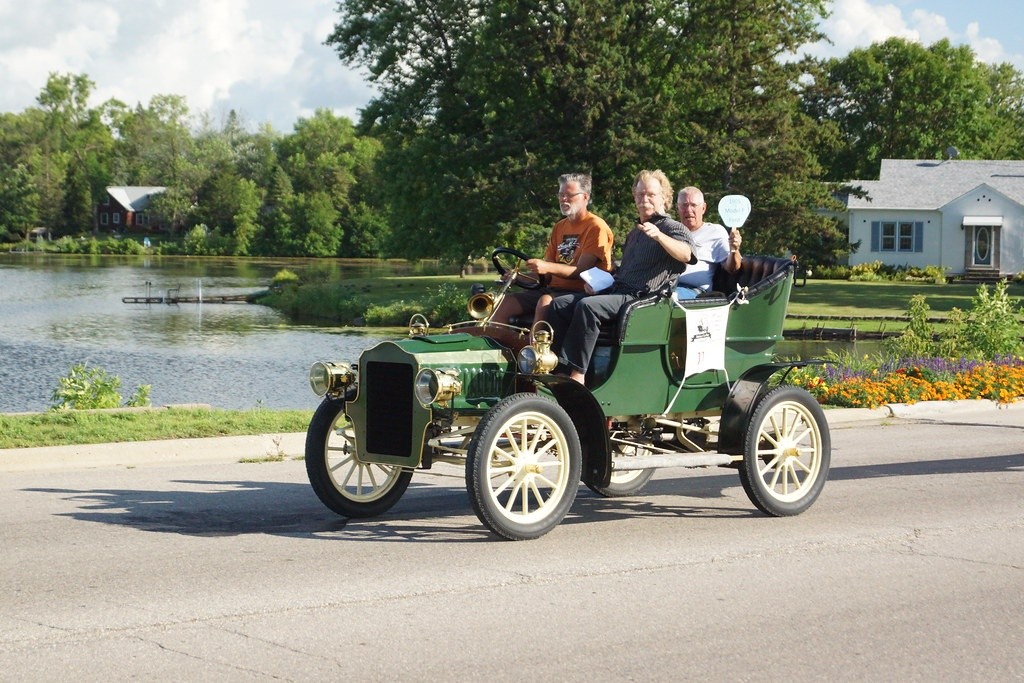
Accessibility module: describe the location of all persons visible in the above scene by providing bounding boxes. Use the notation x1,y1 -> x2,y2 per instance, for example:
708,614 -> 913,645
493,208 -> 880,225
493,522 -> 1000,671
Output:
674,187 -> 742,299
489,173 -> 614,338
545,169 -> 699,386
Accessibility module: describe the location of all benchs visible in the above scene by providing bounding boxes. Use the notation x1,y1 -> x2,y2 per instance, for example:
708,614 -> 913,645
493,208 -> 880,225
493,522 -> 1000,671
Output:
508,274 -> 682,347
712,255 -> 797,300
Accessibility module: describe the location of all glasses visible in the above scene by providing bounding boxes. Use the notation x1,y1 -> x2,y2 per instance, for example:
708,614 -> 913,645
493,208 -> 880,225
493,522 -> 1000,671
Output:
557,193 -> 586,198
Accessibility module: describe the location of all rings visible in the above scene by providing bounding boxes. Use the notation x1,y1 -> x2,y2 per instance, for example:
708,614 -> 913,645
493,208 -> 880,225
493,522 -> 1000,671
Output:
736,238 -> 738,242
